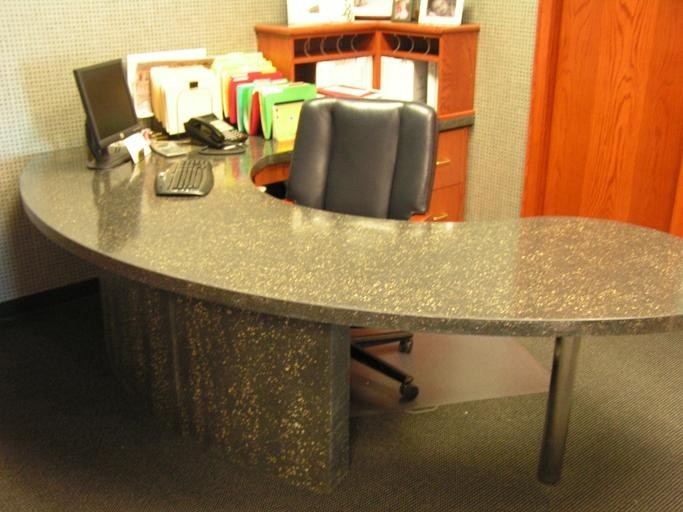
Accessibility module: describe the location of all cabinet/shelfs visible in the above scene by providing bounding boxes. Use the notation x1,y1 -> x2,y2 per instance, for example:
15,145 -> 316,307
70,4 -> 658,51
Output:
255,15 -> 480,117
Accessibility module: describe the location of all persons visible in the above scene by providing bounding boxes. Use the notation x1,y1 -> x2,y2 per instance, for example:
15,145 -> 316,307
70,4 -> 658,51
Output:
427,0 -> 456,18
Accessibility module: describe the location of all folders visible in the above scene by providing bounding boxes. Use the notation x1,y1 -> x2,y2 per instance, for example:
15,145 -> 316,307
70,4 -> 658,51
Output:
208,50 -> 321,141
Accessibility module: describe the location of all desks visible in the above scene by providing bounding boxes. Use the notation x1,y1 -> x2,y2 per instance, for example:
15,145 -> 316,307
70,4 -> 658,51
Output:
18,128 -> 682,493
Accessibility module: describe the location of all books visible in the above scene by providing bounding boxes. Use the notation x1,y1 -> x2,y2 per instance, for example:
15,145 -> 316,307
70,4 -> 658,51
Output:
126,48 -> 383,141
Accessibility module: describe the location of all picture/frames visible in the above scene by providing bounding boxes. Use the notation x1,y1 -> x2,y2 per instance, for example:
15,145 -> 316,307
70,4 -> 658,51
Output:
391,0 -> 465,28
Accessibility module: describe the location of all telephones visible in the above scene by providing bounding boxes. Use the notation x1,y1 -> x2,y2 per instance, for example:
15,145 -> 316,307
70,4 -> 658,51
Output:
184,113 -> 248,149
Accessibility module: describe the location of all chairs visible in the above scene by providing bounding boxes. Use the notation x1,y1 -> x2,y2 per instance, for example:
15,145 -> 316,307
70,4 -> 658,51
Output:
282,95 -> 437,406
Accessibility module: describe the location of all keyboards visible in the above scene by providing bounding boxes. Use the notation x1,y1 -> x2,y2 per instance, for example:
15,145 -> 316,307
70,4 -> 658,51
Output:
155,159 -> 214,196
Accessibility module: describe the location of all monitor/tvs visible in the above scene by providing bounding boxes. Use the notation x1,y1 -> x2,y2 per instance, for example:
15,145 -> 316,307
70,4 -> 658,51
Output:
73,58 -> 143,170
